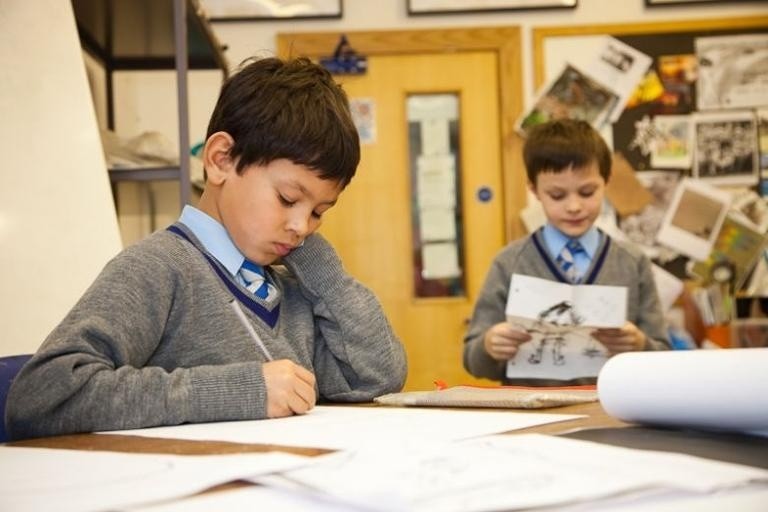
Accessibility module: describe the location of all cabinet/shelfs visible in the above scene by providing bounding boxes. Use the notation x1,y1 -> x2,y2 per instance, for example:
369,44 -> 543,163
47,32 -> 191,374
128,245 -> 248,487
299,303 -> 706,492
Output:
72,0 -> 232,236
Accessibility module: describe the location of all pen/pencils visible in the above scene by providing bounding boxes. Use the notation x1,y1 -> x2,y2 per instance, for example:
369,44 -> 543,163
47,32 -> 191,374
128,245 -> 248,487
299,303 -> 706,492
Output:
228,299 -> 274,362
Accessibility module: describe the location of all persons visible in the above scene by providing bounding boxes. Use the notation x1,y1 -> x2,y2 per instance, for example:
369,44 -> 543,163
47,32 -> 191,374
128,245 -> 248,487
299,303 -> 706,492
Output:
1,40 -> 407,443
462,118 -> 673,387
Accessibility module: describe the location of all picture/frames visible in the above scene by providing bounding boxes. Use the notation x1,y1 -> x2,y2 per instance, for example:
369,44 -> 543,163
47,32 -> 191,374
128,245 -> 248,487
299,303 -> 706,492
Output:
644,0 -> 767,11
196,0 -> 345,24
404,0 -> 580,18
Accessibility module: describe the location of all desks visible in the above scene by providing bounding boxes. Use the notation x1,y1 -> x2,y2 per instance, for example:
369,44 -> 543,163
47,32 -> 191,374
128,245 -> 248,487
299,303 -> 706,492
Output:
0,379 -> 768,511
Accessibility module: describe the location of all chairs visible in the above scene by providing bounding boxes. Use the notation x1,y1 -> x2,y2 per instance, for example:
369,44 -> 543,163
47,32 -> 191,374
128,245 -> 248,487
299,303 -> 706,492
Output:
0,352 -> 34,387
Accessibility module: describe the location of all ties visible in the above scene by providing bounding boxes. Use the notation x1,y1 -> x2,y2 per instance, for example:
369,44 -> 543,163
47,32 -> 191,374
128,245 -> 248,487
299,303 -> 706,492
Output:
554,241 -> 594,284
235,256 -> 282,307
